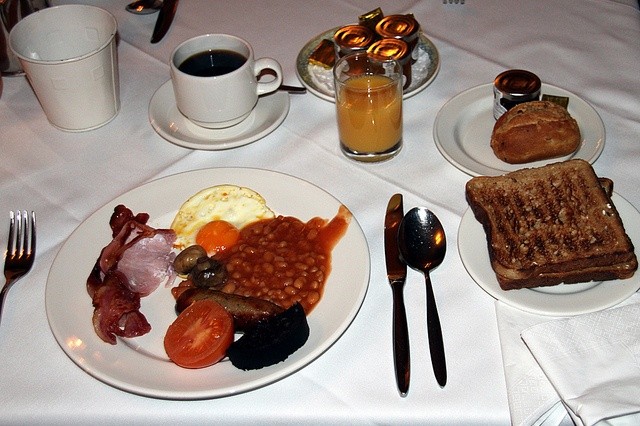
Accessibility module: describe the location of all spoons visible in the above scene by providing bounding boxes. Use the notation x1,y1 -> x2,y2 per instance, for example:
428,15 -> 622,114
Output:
397,206 -> 448,394
125,2 -> 159,16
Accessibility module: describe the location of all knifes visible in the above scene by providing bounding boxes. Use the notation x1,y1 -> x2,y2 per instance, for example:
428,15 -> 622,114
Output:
384,194 -> 411,396
150,1 -> 179,45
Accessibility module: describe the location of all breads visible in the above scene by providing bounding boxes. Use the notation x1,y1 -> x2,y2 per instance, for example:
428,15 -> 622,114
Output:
465,158 -> 634,280
497,175 -> 635,288
492,103 -> 583,165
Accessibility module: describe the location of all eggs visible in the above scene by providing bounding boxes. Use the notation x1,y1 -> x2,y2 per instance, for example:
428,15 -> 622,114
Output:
168,184 -> 276,261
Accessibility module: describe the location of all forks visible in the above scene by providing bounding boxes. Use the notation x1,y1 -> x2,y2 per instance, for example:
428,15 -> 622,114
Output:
1,210 -> 38,320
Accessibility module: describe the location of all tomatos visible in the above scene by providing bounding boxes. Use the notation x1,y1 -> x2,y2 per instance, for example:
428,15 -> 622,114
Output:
163,300 -> 234,369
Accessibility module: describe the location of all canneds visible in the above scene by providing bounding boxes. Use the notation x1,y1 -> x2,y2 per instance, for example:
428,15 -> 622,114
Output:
378,12 -> 421,64
495,68 -> 543,121
366,37 -> 412,90
334,23 -> 376,76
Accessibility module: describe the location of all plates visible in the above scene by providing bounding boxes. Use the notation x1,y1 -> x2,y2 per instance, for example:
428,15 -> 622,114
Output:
458,184 -> 639,317
431,80 -> 606,178
148,77 -> 291,150
44,166 -> 372,400
295,23 -> 440,103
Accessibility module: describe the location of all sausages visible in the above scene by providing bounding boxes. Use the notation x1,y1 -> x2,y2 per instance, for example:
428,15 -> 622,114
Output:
175,288 -> 288,333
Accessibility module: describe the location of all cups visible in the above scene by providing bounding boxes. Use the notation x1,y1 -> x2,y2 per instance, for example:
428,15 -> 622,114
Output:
167,33 -> 283,127
333,52 -> 405,162
8,3 -> 121,132
